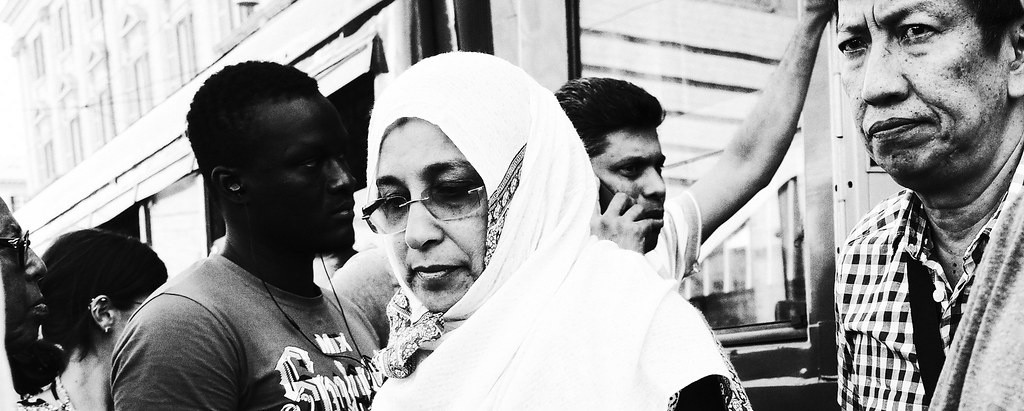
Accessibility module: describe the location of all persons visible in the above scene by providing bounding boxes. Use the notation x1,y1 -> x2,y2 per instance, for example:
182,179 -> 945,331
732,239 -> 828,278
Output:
0,61 -> 399,410
368,50 -> 753,411
834,0 -> 1024,410
556,0 -> 838,291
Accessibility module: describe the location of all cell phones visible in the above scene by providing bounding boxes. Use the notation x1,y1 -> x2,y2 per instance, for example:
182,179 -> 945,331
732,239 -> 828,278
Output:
598,180 -> 644,221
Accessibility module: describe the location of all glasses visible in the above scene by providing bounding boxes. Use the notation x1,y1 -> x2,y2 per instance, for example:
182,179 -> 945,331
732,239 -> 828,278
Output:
0,230 -> 31,270
361,180 -> 487,237
